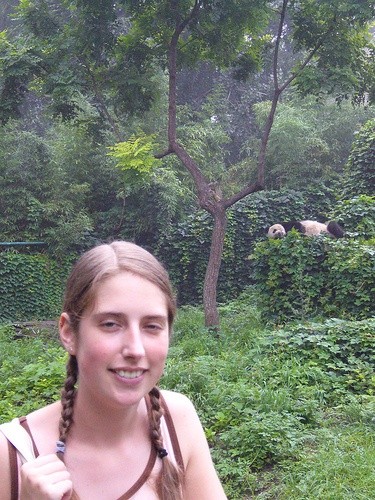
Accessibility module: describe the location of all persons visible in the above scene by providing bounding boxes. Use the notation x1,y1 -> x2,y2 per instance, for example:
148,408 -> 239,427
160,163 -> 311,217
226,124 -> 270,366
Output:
0,240 -> 229,500
